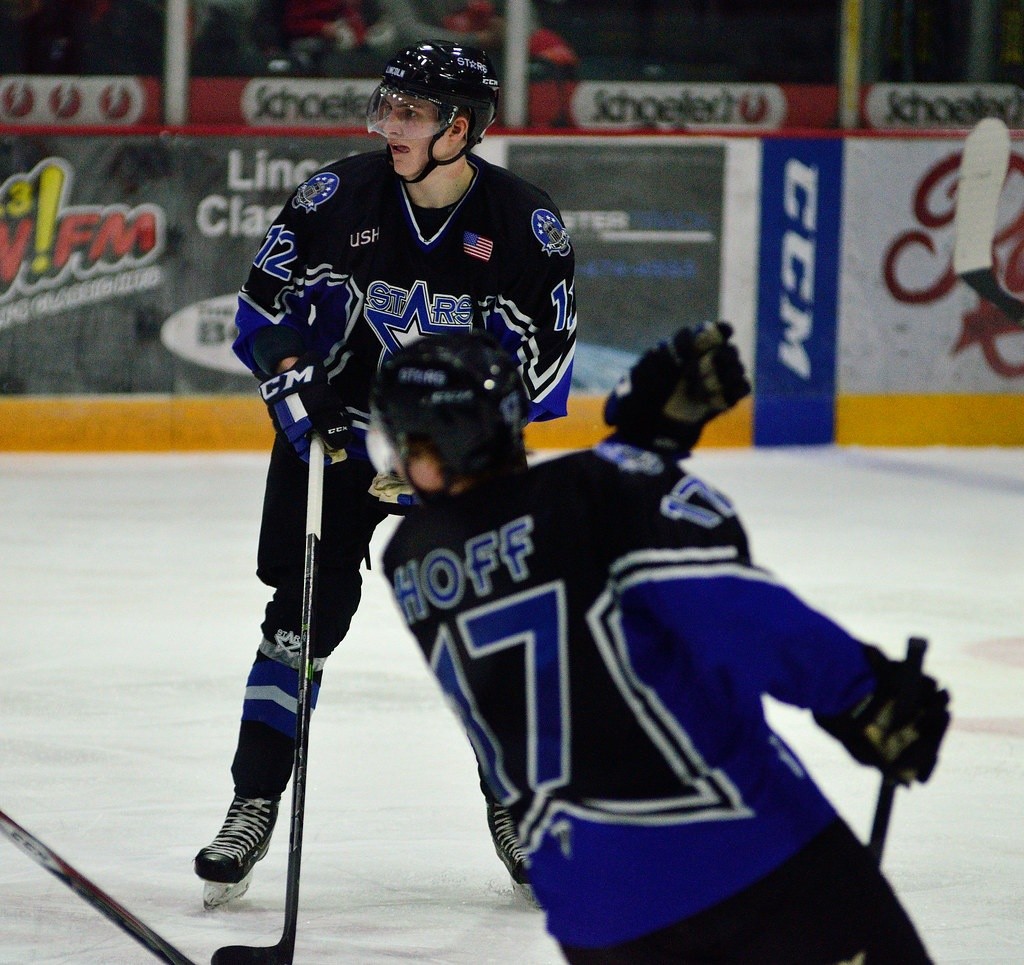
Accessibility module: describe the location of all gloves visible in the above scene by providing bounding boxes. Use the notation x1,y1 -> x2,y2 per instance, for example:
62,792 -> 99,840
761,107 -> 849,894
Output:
604,321 -> 751,457
259,352 -> 353,467
815,647 -> 951,787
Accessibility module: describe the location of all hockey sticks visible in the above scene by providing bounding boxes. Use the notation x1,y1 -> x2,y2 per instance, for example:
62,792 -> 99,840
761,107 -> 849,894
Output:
855,635 -> 933,865
0,811 -> 195,965
208,431 -> 328,965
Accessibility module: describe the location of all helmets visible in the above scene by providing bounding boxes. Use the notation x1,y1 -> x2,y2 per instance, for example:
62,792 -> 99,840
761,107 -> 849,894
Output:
365,38 -> 501,144
366,330 -> 532,479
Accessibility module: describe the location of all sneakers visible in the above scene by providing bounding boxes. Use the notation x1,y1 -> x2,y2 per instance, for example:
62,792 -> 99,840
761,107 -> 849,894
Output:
487,796 -> 545,912
193,793 -> 280,910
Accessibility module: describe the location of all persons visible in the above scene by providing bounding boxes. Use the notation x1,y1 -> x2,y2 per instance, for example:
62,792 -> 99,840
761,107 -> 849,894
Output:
0,1 -> 604,83
376,317 -> 952,963
194,38 -> 579,913
